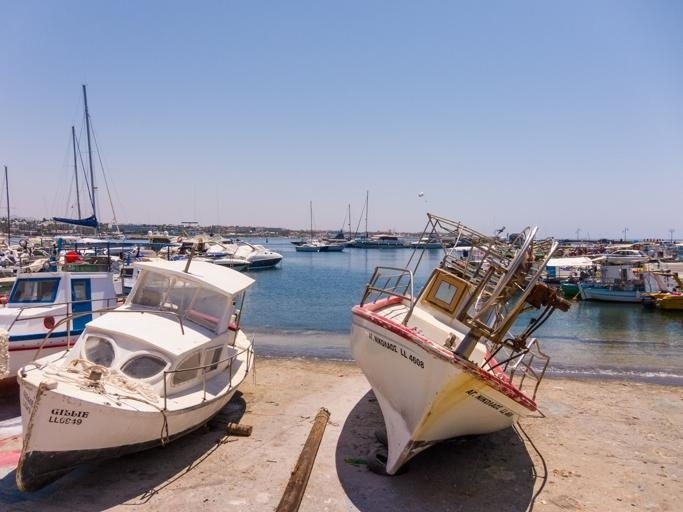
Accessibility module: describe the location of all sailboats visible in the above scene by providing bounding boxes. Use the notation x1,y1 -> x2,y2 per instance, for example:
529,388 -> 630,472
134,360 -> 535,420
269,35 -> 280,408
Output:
289,188 -> 399,252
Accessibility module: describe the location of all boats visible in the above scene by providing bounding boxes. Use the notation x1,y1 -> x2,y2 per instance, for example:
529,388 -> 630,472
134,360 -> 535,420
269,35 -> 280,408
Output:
349,211 -> 571,477
412,232 -> 683,314
